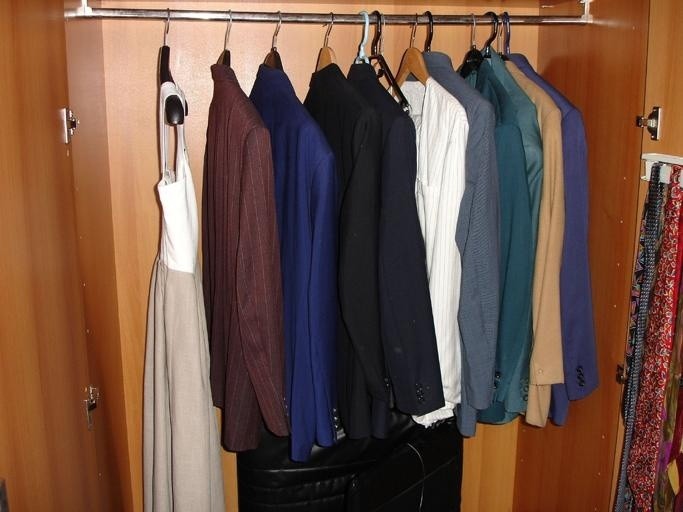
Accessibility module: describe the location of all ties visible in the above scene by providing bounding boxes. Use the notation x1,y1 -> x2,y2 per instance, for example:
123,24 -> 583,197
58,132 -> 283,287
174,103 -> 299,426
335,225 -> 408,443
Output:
615,165 -> 682,512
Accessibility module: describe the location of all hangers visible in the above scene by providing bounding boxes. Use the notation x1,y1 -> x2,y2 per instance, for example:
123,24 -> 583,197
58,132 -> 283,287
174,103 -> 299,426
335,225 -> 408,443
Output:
207,8 -> 512,127
153,7 -> 191,127
